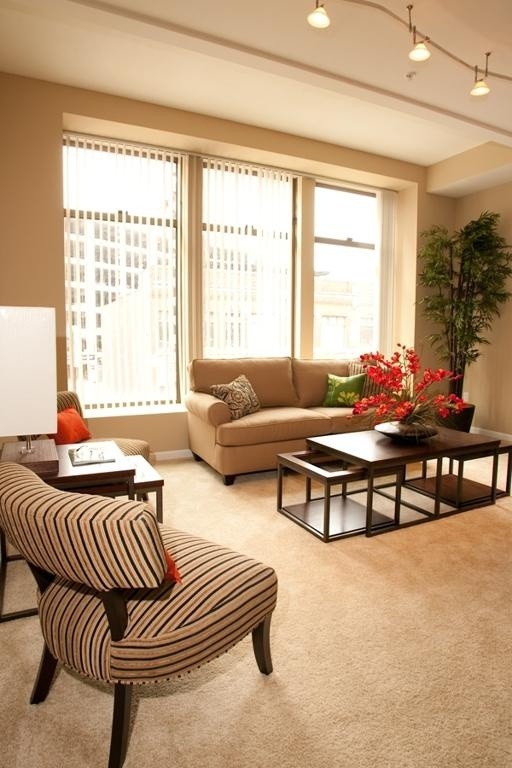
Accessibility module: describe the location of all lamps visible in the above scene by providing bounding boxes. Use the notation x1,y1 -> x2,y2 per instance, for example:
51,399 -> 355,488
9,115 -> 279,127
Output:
1,306 -> 59,473
307,1 -> 329,29
408,27 -> 431,61
470,66 -> 491,96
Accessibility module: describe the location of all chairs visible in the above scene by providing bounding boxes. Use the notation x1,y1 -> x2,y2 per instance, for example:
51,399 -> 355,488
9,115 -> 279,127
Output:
0,460 -> 279,766
17,391 -> 150,459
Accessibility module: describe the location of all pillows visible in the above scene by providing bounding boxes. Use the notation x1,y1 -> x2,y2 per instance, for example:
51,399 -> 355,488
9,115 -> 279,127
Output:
348,363 -> 397,407
321,372 -> 366,407
47,402 -> 94,444
210,374 -> 261,420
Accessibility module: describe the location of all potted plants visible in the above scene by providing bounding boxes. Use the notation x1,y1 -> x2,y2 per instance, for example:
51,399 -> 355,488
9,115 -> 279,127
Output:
413,209 -> 511,433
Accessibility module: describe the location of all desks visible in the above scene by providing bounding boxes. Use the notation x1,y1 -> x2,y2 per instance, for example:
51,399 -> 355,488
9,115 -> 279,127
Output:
0,439 -> 136,624
67,454 -> 164,521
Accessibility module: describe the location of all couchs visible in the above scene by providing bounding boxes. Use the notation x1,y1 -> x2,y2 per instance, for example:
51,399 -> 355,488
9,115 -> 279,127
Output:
182,356 -> 410,485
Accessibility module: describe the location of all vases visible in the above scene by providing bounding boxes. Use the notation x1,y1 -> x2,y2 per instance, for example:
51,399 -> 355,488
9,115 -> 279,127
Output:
374,422 -> 439,448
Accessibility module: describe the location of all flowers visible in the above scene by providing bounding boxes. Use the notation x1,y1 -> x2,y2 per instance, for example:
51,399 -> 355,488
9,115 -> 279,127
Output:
345,343 -> 469,444
